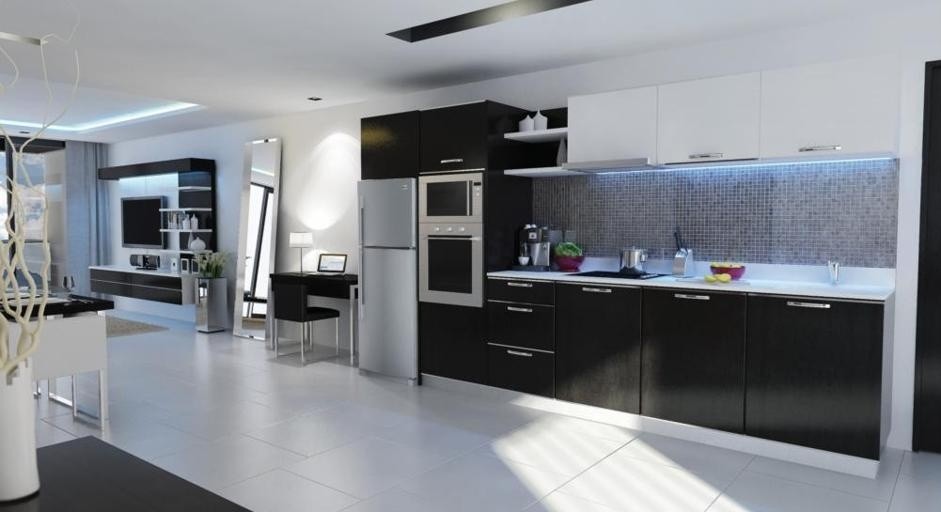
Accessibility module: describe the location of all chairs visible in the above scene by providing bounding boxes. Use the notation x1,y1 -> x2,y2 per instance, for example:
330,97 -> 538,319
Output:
270,279 -> 340,364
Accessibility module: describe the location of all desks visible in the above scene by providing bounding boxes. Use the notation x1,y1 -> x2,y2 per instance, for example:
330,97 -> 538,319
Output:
268,269 -> 360,363
0,434 -> 255,512
1,282 -> 116,446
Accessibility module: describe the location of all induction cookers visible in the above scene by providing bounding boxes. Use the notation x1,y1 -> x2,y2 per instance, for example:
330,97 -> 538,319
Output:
565,270 -> 672,281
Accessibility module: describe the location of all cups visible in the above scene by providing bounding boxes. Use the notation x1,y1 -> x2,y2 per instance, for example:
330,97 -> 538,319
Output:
170,256 -> 178,273
828,262 -> 840,284
518,257 -> 530,266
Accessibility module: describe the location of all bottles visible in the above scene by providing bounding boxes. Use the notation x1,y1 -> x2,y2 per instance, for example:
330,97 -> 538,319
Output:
533,107 -> 548,131
188,233 -> 195,251
518,114 -> 535,133
168,212 -> 198,229
556,137 -> 567,166
191,237 -> 207,252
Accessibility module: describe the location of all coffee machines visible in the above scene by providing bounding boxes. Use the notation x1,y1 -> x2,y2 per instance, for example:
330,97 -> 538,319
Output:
519,227 -> 550,271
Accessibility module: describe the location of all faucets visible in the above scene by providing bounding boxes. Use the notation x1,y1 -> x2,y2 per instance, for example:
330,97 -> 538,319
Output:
833,261 -> 841,282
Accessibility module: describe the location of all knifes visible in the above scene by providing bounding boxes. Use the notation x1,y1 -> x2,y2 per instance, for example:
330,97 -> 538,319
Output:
675,226 -> 689,255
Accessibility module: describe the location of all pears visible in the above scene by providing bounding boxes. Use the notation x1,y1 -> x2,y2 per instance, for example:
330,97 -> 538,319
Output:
704,263 -> 742,282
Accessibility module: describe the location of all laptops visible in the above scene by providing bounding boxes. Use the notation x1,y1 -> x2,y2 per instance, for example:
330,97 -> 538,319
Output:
306,253 -> 347,276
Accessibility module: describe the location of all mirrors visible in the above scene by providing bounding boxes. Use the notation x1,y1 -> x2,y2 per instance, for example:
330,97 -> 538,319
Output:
229,134 -> 285,343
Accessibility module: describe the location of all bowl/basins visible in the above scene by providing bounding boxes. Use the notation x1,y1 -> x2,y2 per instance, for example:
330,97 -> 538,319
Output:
553,256 -> 585,273
711,266 -> 746,281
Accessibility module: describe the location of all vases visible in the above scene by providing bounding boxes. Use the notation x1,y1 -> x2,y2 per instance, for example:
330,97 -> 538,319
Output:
193,275 -> 228,335
180,213 -> 207,251
1,353 -> 43,506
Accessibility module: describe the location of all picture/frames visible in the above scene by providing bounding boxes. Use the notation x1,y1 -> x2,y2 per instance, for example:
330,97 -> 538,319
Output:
317,251 -> 349,273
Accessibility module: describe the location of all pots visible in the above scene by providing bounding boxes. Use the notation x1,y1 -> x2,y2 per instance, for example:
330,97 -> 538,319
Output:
619,245 -> 649,276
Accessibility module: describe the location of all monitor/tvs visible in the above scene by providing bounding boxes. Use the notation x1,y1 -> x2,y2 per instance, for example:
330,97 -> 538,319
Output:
120,195 -> 166,250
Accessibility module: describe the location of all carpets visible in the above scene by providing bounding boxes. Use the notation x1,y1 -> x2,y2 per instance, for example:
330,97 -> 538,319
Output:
104,312 -> 169,340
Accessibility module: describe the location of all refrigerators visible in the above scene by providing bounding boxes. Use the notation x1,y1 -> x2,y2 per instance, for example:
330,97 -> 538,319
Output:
358,176 -> 418,378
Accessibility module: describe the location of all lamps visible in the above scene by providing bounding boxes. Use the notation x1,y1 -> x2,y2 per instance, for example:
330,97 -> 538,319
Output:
288,230 -> 316,274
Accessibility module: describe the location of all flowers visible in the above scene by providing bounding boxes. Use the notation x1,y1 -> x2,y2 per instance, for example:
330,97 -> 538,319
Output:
190,249 -> 229,281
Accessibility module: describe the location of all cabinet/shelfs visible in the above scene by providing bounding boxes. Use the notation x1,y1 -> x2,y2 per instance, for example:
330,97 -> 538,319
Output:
85,263 -> 197,307
636,276 -> 750,449
551,273 -> 642,424
480,264 -> 582,409
743,280 -> 899,474
155,182 -> 215,256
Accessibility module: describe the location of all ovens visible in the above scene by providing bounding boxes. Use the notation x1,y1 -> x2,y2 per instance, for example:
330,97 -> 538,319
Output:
416,171 -> 486,311
